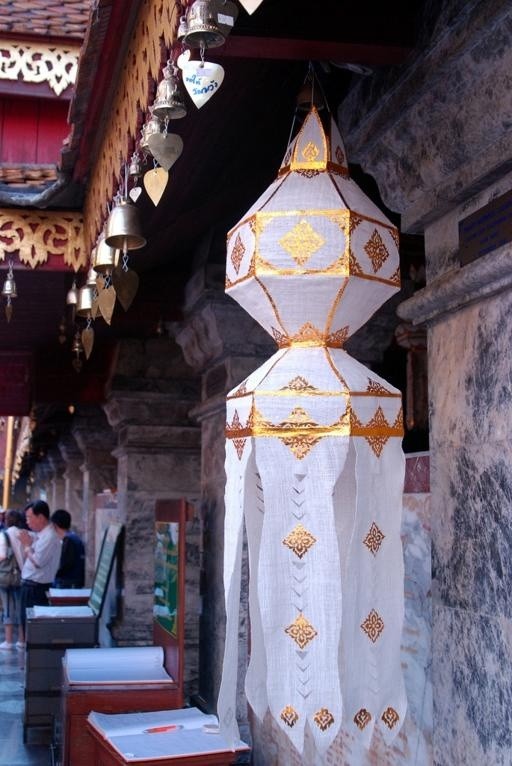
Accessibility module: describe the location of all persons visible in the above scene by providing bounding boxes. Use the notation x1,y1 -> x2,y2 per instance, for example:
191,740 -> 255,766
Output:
0,498 -> 37,649
18,500 -> 60,639
51,510 -> 85,589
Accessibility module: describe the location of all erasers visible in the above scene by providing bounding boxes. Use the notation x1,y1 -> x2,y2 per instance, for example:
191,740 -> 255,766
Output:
203,725 -> 219,734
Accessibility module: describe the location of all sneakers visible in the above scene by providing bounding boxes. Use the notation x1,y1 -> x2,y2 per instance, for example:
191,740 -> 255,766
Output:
0,639 -> 27,650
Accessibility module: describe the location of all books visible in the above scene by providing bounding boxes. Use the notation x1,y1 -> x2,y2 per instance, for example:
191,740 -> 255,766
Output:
61,645 -> 175,685
88,706 -> 253,762
49,588 -> 91,597
33,605 -> 94,619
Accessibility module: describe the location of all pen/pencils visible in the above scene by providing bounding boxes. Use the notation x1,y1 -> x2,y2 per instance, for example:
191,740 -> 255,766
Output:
143,726 -> 183,733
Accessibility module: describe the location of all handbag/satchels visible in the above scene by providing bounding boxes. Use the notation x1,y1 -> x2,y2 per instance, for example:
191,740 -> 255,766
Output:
0,554 -> 21,589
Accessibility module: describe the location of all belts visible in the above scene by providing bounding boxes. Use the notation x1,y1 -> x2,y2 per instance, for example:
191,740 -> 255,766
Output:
20,579 -> 36,586
